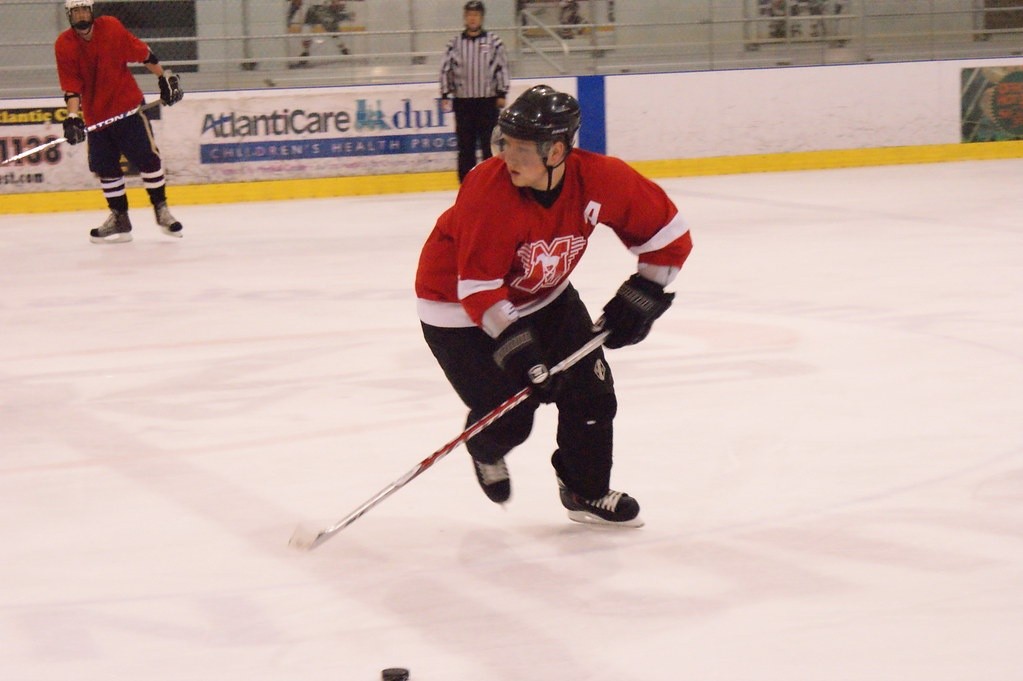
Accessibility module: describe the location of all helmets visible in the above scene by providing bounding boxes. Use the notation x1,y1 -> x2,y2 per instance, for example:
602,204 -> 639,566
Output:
463,2 -> 485,15
500,84 -> 582,143
64,0 -> 95,12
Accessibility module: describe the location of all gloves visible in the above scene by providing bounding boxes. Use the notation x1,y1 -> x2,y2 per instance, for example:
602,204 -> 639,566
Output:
491,319 -> 567,410
62,116 -> 85,144
594,274 -> 675,350
159,69 -> 184,107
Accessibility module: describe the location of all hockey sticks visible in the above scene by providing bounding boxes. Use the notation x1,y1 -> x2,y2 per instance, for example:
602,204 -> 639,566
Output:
0,98 -> 165,166
289,330 -> 614,553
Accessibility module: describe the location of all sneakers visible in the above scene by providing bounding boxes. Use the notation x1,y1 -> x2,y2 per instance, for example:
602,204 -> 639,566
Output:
551,451 -> 645,529
153,201 -> 182,237
90,208 -> 134,244
469,452 -> 512,513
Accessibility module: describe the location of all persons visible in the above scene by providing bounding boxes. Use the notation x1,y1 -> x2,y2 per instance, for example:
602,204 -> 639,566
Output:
411,84 -> 698,527
54,0 -> 187,241
434,0 -> 512,184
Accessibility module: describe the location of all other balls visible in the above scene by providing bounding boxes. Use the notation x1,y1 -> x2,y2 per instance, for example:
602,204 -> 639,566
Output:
382,669 -> 410,681
